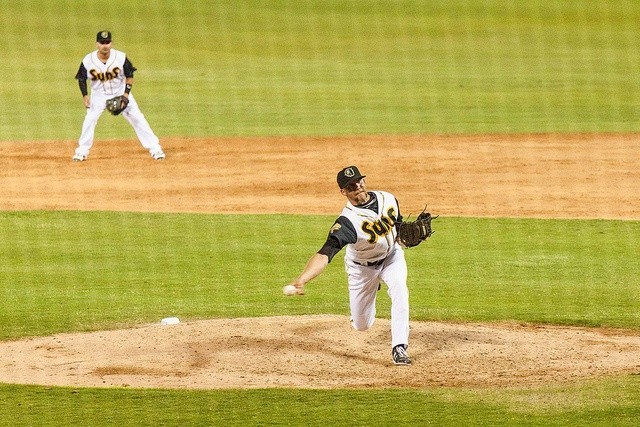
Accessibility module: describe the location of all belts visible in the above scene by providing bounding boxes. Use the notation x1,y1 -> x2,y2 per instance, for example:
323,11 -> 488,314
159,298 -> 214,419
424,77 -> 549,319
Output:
353,258 -> 387,267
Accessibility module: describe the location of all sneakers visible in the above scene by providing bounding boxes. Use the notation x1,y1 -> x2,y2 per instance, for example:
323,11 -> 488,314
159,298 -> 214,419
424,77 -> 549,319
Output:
392,345 -> 412,365
73,153 -> 88,161
153,152 -> 166,160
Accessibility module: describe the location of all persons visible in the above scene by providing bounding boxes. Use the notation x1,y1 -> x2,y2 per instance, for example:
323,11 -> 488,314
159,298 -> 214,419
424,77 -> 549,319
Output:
281,165 -> 439,366
72,30 -> 166,162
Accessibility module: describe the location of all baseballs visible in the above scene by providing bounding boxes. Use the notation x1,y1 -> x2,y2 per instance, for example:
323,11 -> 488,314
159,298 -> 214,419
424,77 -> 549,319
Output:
282,284 -> 297,295
161,316 -> 180,326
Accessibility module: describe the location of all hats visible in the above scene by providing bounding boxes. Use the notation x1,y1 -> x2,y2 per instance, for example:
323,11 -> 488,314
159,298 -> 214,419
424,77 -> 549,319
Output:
337,165 -> 366,188
97,30 -> 112,41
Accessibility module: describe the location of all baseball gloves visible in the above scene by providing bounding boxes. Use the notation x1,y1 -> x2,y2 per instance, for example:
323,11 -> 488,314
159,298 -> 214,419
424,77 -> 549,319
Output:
105,95 -> 129,116
394,205 -> 439,248
